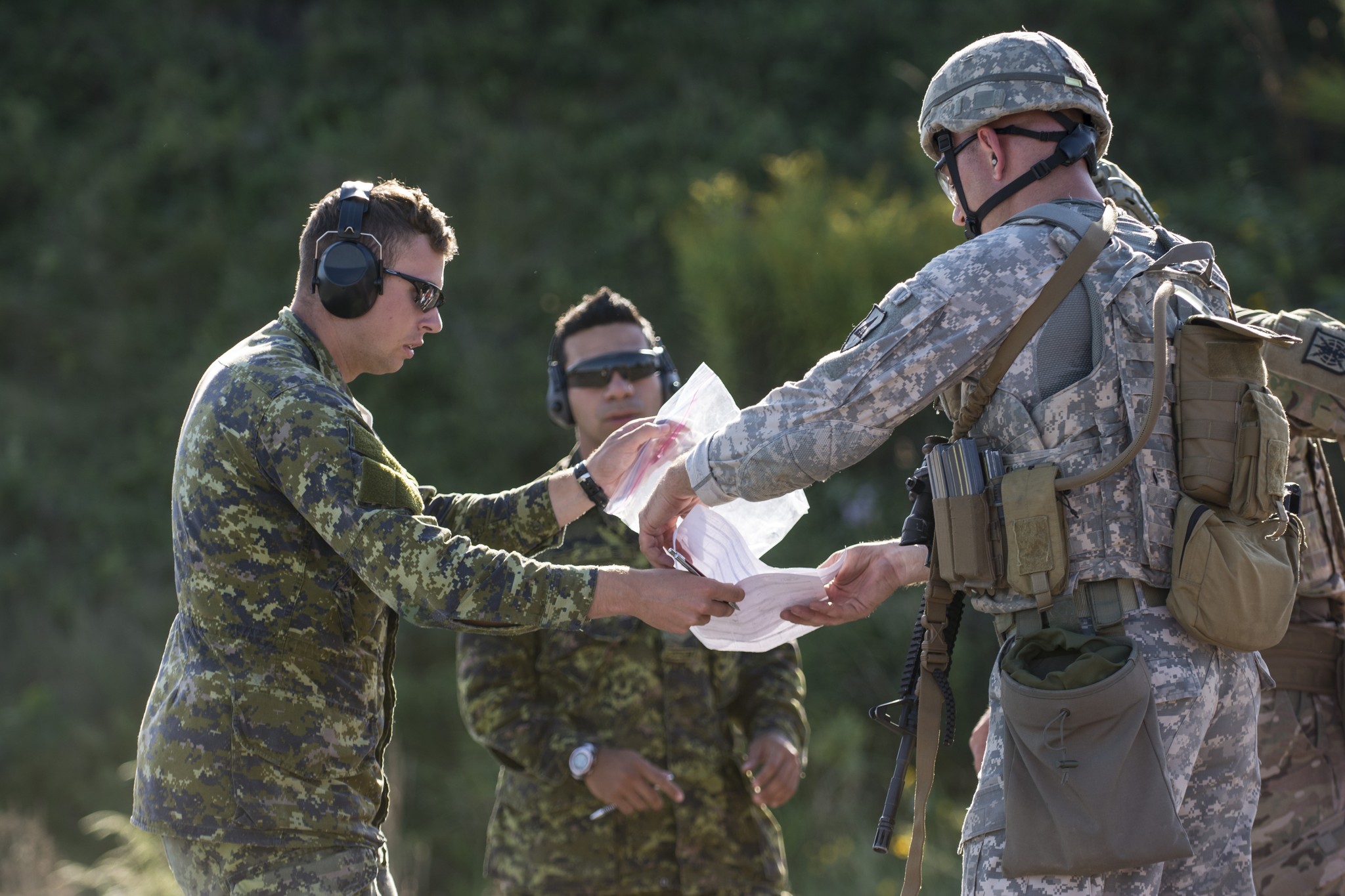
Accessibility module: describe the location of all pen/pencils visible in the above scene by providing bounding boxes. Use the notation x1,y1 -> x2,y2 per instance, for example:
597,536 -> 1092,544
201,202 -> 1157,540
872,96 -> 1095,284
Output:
588,773 -> 673,822
668,547 -> 743,612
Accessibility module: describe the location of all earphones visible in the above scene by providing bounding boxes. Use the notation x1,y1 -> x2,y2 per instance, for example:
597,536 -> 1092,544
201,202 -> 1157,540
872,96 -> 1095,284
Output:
991,153 -> 998,167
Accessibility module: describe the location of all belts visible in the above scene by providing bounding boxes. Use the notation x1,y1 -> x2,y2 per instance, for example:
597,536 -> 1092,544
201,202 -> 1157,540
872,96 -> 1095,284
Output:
991,571 -> 1172,652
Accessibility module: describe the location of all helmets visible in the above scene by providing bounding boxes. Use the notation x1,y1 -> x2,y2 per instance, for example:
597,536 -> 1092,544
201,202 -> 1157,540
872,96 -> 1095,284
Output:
917,29 -> 1114,167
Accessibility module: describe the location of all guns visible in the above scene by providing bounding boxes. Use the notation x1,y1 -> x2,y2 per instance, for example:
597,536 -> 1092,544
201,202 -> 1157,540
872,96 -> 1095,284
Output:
869,435 -> 964,857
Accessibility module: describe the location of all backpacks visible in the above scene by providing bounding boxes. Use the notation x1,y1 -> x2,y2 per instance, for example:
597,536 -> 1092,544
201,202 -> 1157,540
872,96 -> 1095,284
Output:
1000,202 -> 1345,652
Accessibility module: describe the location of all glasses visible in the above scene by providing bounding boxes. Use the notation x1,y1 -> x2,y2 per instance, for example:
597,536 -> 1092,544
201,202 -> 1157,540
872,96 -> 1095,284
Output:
382,267 -> 447,313
934,131 -> 978,207
560,346 -> 663,389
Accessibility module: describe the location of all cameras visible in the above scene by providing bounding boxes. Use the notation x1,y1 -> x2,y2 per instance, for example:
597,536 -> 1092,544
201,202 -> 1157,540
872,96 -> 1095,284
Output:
1284,482 -> 1302,516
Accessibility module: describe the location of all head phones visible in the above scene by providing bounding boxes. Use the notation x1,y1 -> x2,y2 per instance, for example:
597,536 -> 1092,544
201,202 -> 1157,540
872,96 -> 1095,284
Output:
546,302 -> 681,429
311,182 -> 384,318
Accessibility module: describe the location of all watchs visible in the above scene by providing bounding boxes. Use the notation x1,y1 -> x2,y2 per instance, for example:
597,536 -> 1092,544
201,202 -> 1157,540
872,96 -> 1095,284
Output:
568,742 -> 599,786
574,458 -> 609,508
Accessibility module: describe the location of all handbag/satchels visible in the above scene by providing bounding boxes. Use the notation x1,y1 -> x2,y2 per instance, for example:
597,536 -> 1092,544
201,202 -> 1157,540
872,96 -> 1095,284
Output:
1001,628 -> 1197,879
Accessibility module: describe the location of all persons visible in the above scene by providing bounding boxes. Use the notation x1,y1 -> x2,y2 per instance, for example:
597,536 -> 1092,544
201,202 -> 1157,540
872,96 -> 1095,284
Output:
129,184 -> 743,895
453,285 -> 811,896
639,32 -> 1295,896
965,300 -> 1344,896
